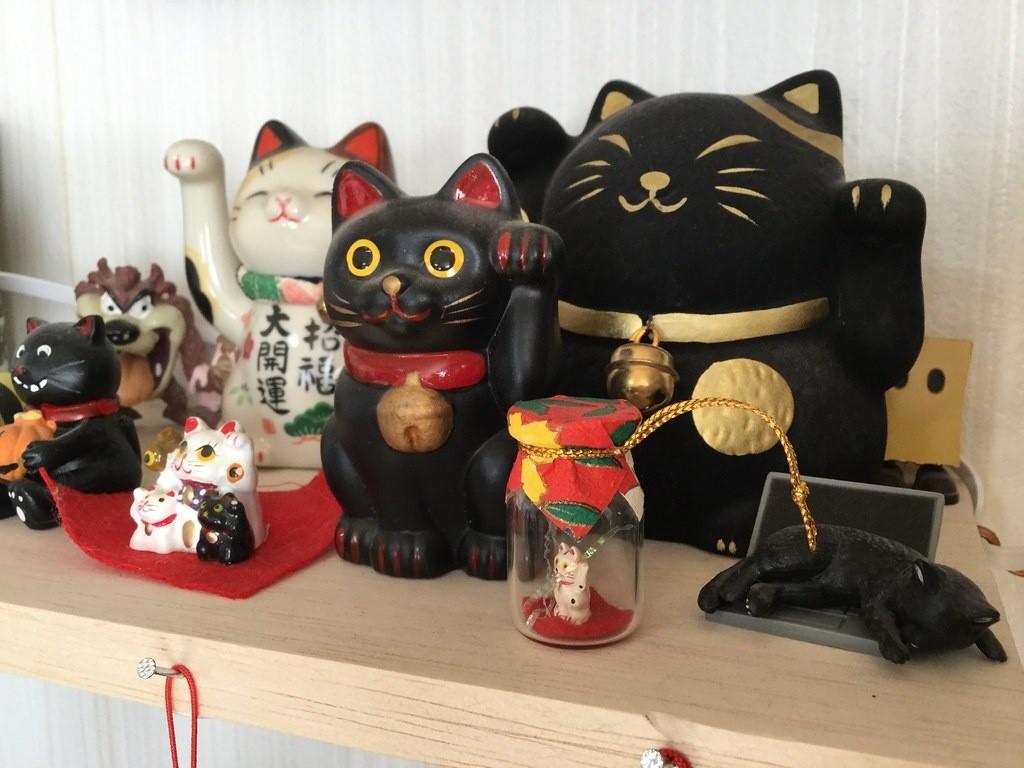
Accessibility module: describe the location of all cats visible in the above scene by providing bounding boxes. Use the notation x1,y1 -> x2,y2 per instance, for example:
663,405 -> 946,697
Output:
196,490 -> 254,565
0,314 -> 144,530
697,522 -> 1010,665
129,486 -> 202,555
153,415 -> 271,549
163,118 -> 397,472
485,69 -> 928,557
320,152 -> 567,579
552,542 -> 591,625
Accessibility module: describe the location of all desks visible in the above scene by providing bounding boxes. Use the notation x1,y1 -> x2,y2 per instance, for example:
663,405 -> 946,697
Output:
0,464 -> 1024,768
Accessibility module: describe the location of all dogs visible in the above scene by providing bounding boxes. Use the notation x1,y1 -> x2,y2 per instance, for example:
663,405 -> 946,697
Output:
73,257 -> 223,429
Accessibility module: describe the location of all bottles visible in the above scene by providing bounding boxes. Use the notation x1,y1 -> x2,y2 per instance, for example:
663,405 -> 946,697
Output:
506,395 -> 640,642
